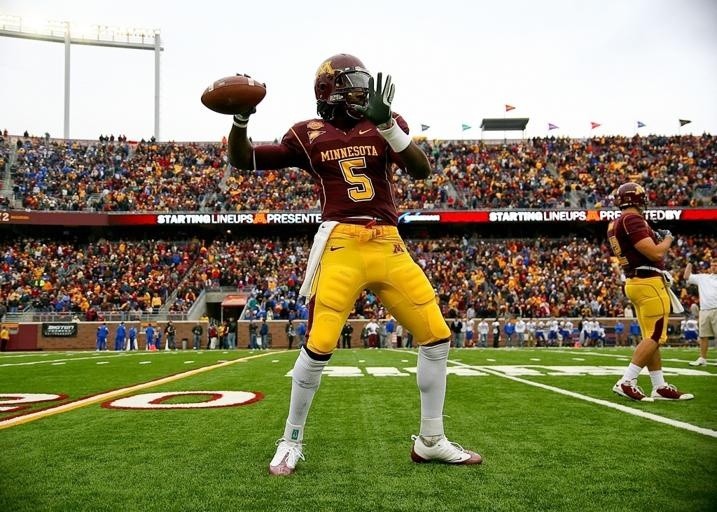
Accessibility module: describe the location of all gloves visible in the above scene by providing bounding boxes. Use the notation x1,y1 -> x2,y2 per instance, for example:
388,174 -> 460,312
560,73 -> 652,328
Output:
687,253 -> 698,264
348,68 -> 396,130
654,229 -> 675,243
231,70 -> 268,130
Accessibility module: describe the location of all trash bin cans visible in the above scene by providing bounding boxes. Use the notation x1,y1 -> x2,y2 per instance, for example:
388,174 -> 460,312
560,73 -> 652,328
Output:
182,338 -> 188,350
256,336 -> 261,345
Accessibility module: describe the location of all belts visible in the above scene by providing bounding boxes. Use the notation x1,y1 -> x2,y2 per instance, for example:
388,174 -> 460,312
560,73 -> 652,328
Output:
332,224 -> 399,242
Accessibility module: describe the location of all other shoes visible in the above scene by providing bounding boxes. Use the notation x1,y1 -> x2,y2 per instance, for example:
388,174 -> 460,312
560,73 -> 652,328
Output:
452,344 -> 633,348
221,348 -> 270,351
95,346 -> 196,351
364,346 -> 413,349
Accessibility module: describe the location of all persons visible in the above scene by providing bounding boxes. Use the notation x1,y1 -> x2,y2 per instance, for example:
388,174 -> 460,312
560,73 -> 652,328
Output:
0,129 -> 717,214
684,253 -> 717,367
607,182 -> 694,403
1,234 -> 717,351
227,54 -> 483,474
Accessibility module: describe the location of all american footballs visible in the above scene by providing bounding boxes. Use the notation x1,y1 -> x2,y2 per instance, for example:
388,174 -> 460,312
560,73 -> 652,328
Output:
200,76 -> 266,114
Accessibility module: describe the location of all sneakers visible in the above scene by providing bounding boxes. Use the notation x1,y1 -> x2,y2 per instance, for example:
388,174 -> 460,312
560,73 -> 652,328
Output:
651,382 -> 695,402
611,377 -> 655,403
688,357 -> 710,366
409,433 -> 482,466
267,434 -> 306,478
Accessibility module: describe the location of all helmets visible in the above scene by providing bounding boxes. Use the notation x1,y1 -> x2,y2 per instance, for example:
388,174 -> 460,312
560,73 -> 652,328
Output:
614,182 -> 649,211
313,53 -> 374,124
